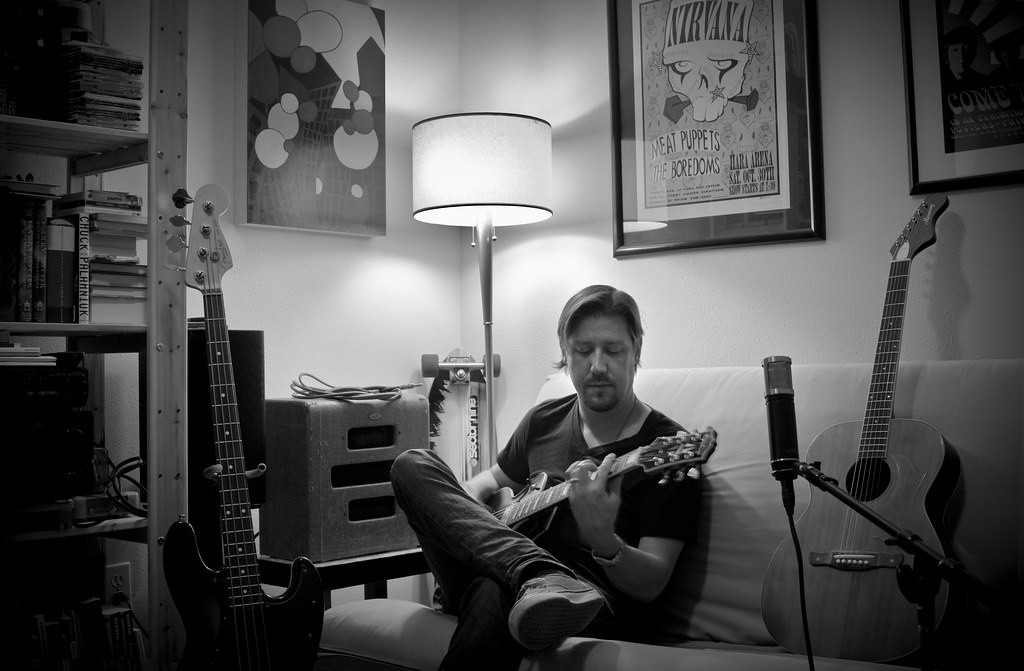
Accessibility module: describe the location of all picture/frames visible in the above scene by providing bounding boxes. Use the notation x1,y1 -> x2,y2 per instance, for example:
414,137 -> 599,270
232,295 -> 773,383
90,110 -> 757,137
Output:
899,0 -> 1024,197
604,0 -> 827,258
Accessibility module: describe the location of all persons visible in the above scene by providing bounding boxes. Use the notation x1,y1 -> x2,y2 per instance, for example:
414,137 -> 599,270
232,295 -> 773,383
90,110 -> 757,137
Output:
390,285 -> 705,671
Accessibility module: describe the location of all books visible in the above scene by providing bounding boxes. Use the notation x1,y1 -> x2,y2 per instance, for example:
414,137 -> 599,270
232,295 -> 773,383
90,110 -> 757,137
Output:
20,597 -> 147,671
0,331 -> 68,366
61,30 -> 144,131
0,181 -> 149,325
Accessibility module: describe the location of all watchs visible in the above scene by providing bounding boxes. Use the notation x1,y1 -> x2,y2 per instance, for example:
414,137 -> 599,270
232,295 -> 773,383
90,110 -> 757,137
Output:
592,533 -> 625,566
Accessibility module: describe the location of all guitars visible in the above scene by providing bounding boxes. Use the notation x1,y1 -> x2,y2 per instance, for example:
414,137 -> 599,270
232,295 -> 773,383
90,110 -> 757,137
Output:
161,181 -> 323,671
486,424 -> 718,527
761,192 -> 963,660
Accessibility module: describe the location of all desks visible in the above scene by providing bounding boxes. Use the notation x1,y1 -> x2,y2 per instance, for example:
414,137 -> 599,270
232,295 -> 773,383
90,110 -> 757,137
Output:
255,545 -> 431,612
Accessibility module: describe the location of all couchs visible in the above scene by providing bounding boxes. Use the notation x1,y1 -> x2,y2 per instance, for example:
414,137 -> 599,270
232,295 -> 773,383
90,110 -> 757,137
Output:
316,358 -> 1024,671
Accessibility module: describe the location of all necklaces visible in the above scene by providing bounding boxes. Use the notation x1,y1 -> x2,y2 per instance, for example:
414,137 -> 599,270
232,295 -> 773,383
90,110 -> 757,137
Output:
615,395 -> 637,441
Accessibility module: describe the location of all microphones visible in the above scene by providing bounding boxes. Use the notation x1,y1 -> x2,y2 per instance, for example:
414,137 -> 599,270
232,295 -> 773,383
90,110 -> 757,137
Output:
762,356 -> 800,515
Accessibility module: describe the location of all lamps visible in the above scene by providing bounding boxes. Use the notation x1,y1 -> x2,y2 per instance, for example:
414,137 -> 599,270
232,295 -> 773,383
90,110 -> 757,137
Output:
410,111 -> 552,474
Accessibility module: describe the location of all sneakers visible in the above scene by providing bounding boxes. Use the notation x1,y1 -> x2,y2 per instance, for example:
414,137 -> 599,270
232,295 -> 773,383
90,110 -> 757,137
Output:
507,569 -> 605,650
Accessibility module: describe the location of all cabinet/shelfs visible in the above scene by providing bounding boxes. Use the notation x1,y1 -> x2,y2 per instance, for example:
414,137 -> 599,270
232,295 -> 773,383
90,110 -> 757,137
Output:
0,0 -> 189,671
